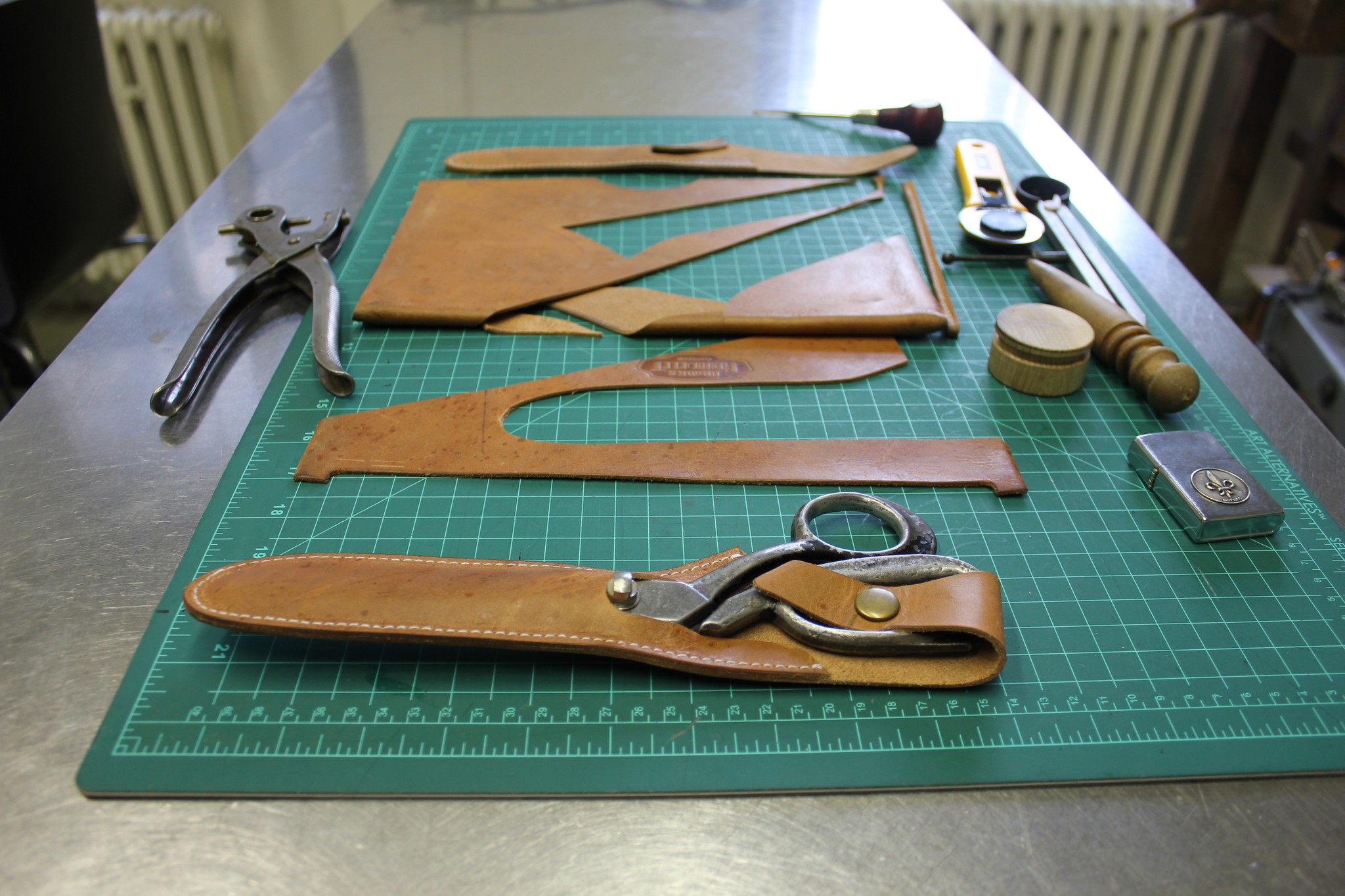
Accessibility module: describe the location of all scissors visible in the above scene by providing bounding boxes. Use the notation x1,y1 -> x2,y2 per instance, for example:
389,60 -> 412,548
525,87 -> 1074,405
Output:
182,490 -> 992,663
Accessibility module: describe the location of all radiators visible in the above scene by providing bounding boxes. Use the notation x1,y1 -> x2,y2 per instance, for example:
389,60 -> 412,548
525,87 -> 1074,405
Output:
944,0 -> 1226,247
39,6 -> 236,314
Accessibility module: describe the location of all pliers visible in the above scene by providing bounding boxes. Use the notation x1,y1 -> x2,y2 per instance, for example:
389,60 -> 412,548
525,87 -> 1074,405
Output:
147,200 -> 357,419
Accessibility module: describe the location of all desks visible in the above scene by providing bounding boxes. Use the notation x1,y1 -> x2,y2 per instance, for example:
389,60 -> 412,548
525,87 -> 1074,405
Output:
0,0 -> 1345,896
